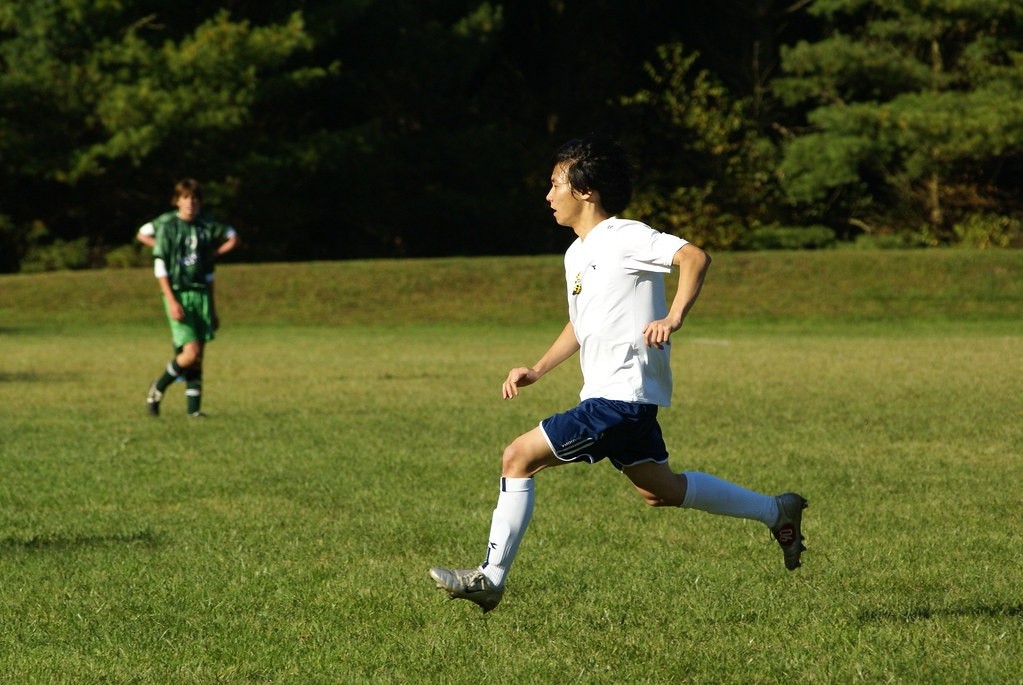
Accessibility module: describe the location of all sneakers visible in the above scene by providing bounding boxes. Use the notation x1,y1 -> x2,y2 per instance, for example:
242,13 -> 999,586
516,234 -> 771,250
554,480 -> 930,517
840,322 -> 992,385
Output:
147,382 -> 162,417
429,567 -> 506,614
191,411 -> 209,420
770,493 -> 809,571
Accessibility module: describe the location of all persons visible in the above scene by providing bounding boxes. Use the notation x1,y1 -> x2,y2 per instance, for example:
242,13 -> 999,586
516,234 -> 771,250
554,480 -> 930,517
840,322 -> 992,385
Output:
136,177 -> 236,420
429,144 -> 809,613
